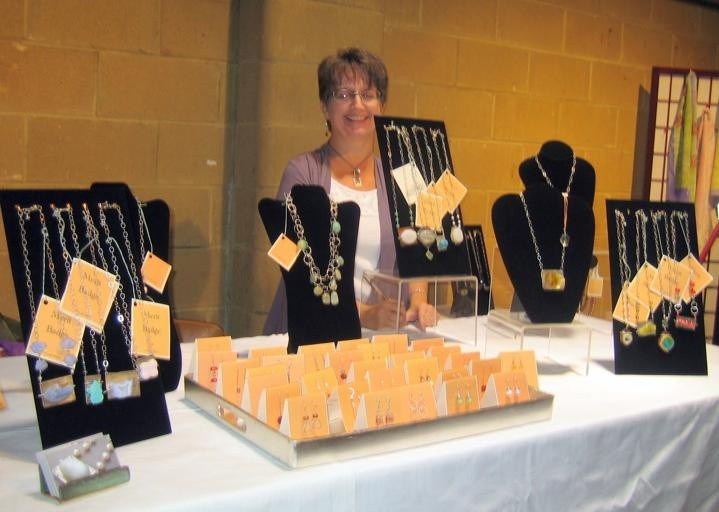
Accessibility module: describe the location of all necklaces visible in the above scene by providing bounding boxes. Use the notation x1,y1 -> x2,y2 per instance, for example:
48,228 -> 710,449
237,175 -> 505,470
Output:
616,208 -> 700,353
282,191 -> 344,308
534,151 -> 578,195
327,143 -> 375,188
17,201 -> 155,406
382,120 -> 491,297
518,193 -> 571,292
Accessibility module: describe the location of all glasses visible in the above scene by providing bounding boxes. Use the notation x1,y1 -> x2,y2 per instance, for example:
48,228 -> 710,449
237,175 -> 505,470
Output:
327,88 -> 382,102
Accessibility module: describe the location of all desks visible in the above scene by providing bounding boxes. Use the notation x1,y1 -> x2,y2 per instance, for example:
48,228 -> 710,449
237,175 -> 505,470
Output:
0,310 -> 719,512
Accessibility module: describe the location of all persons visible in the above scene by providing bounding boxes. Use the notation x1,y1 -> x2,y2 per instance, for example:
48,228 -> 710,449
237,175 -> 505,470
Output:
260,45 -> 443,336
